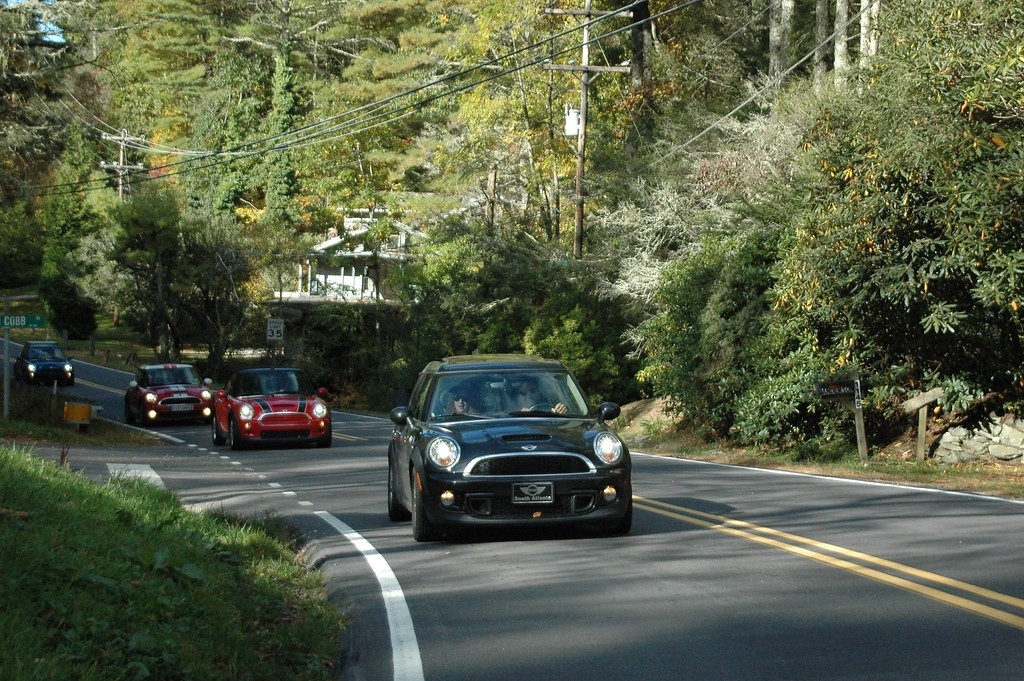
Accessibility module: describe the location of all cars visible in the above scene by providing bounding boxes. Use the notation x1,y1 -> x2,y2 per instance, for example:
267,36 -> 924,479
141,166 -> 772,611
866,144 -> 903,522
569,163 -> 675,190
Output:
211,368 -> 332,446
14,341 -> 74,388
124,363 -> 215,427
387,352 -> 634,541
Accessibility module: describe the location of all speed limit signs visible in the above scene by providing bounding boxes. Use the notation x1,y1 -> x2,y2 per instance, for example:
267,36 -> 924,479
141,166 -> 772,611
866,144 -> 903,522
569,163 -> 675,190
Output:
266,319 -> 284,339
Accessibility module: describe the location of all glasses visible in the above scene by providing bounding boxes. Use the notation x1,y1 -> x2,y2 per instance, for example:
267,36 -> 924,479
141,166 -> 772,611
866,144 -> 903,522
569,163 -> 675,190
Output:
520,387 -> 536,395
453,395 -> 470,402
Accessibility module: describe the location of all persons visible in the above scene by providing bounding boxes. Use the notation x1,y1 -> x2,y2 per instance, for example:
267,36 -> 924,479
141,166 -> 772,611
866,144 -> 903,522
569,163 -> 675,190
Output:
445,384 -> 480,415
506,377 -> 568,415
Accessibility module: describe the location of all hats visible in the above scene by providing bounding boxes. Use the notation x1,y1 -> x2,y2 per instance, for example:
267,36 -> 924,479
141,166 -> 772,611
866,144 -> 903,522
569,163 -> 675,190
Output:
449,384 -> 472,394
